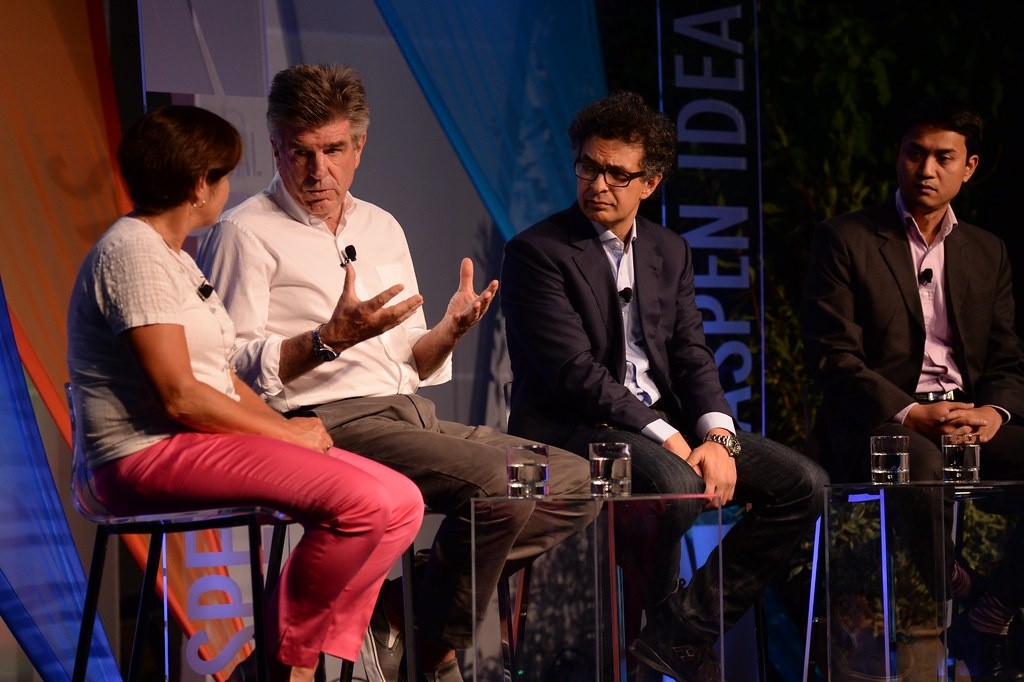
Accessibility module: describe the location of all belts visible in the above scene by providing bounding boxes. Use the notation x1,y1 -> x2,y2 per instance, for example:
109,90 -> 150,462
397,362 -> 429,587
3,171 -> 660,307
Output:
914,389 -> 965,403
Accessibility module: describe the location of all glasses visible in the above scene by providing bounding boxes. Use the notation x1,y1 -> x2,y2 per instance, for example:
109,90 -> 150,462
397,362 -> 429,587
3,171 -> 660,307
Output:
573,158 -> 646,187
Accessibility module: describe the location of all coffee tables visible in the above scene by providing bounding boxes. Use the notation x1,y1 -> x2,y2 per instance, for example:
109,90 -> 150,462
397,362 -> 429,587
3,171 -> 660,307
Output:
473,493 -> 726,682
823,481 -> 1024,682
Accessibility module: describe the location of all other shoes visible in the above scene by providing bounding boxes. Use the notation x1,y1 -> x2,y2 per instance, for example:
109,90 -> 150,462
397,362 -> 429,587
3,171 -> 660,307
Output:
628,621 -> 724,681
423,658 -> 465,682
369,579 -> 405,682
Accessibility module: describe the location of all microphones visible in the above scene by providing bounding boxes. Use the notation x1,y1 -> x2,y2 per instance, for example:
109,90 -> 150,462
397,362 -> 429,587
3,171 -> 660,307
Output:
198,284 -> 213,299
621,288 -> 632,303
923,268 -> 934,283
345,245 -> 356,261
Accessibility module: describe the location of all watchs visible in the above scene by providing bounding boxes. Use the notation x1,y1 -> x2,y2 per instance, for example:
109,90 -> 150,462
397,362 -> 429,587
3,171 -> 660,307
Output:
313,323 -> 340,362
703,434 -> 741,456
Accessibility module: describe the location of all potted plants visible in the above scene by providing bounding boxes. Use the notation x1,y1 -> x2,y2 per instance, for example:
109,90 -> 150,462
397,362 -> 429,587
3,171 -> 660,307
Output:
831,498 -> 1007,682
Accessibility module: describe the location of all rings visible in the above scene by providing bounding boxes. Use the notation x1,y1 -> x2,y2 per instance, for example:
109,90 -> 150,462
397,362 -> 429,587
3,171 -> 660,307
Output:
324,444 -> 330,452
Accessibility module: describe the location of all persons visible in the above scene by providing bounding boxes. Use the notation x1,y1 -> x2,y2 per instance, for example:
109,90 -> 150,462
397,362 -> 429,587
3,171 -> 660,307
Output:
195,63 -> 603,682
810,100 -> 1023,682
66,104 -> 425,682
498,95 -> 829,682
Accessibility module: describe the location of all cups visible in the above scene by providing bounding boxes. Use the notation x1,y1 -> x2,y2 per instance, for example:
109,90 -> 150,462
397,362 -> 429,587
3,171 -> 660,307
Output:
505,444 -> 549,496
942,434 -> 979,481
870,436 -> 909,485
589,443 -> 631,497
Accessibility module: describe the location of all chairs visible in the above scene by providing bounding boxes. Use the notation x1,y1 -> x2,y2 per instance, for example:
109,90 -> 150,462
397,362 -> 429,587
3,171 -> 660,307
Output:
63,380 -> 298,682
